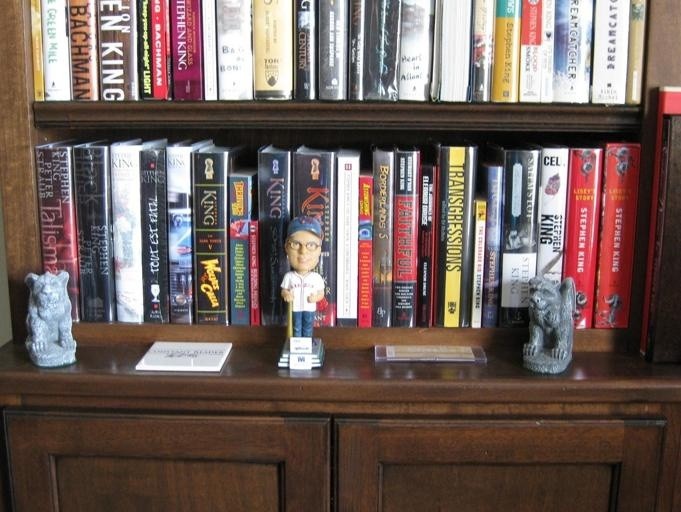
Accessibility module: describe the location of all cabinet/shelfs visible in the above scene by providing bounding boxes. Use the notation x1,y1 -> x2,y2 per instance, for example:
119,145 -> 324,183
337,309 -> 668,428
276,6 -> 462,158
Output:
0,0 -> 681,512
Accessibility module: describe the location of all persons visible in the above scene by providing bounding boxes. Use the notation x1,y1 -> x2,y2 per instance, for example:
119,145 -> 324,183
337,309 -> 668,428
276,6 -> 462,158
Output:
278,214 -> 328,351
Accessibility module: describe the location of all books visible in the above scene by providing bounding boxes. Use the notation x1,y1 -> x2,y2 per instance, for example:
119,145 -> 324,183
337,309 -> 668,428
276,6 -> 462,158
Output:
373,345 -> 489,364
136,340 -> 234,374
641,114 -> 681,360
638,83 -> 681,356
30,0 -> 647,105
37,132 -> 641,329
